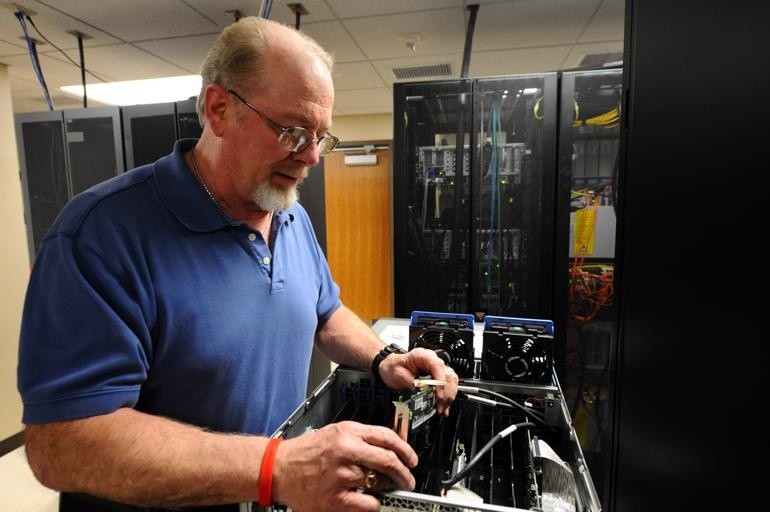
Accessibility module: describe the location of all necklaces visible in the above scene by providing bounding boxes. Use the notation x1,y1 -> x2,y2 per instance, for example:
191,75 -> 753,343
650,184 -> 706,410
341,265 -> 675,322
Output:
187,148 -> 275,240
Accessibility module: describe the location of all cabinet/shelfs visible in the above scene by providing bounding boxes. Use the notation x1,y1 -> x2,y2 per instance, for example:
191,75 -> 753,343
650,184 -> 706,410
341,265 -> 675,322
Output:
299,156 -> 327,262
391,68 -> 627,511
13,101 -> 202,267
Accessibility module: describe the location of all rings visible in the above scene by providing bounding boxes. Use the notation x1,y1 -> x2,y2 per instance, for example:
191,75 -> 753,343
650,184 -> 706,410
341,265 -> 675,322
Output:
363,466 -> 379,491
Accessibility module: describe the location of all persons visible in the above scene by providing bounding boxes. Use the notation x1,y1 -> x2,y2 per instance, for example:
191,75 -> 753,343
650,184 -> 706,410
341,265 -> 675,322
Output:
14,14 -> 461,512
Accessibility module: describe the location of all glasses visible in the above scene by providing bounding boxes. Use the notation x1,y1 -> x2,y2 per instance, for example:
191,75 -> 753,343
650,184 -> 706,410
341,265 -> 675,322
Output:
225,87 -> 339,157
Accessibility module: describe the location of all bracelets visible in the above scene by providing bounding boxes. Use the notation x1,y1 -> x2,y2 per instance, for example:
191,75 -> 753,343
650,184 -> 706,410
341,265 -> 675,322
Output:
258,435 -> 286,510
371,342 -> 407,391
